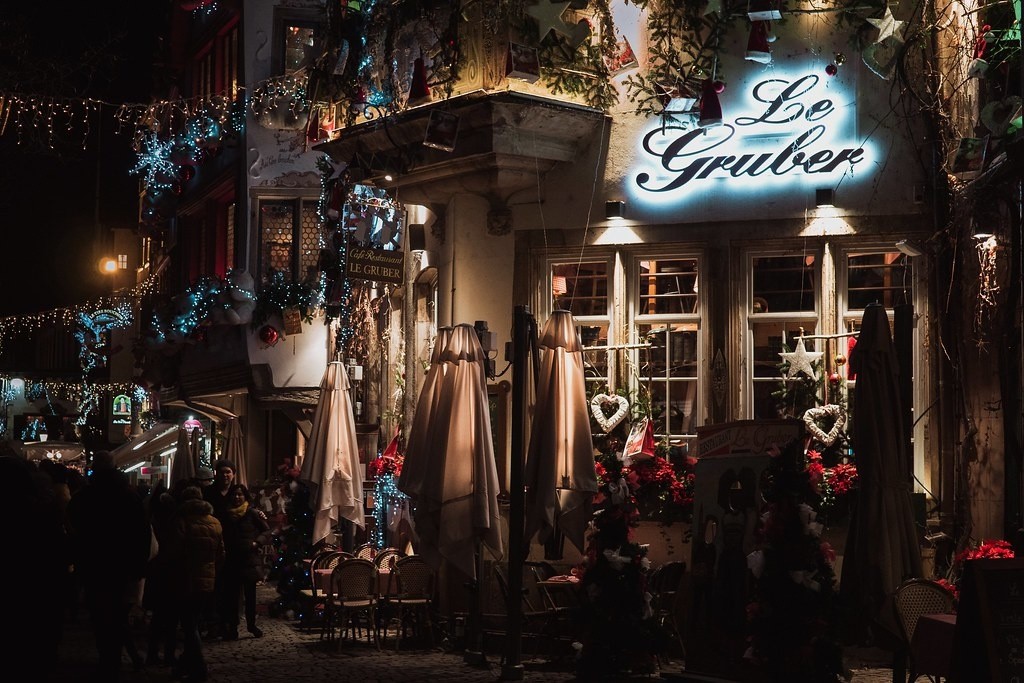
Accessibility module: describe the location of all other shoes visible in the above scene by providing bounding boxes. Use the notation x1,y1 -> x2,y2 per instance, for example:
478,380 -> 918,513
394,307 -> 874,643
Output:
180,671 -> 208,683
135,659 -> 145,672
145,656 -> 164,667
248,626 -> 262,637
163,657 -> 180,665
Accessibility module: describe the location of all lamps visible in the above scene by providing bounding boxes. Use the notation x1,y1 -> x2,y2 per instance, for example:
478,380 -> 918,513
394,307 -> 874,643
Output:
606,202 -> 627,219
816,188 -> 837,210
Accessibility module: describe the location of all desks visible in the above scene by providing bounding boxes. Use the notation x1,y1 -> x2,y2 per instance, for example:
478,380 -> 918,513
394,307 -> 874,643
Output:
315,567 -> 397,641
535,577 -> 589,667
303,556 -> 374,635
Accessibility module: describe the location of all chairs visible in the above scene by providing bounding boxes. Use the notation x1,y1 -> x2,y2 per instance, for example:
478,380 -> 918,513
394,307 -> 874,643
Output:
327,559 -> 383,650
372,548 -> 410,637
891,578 -> 960,683
380,554 -> 434,648
321,552 -> 364,638
300,543 -> 337,633
647,558 -> 686,669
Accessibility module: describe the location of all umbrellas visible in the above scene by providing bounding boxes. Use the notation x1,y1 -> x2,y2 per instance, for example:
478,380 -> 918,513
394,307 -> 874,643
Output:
494,309 -> 598,555
171,427 -> 200,492
841,302 -> 925,640
299,361 -> 365,549
397,323 -> 504,608
221,419 -> 248,490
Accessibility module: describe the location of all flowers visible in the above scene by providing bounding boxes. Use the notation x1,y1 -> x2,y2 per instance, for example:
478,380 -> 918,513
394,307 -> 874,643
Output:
593,444 -> 1015,603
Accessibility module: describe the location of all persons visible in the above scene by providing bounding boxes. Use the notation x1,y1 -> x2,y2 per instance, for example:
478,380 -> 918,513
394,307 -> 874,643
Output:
0,440 -> 271,683
